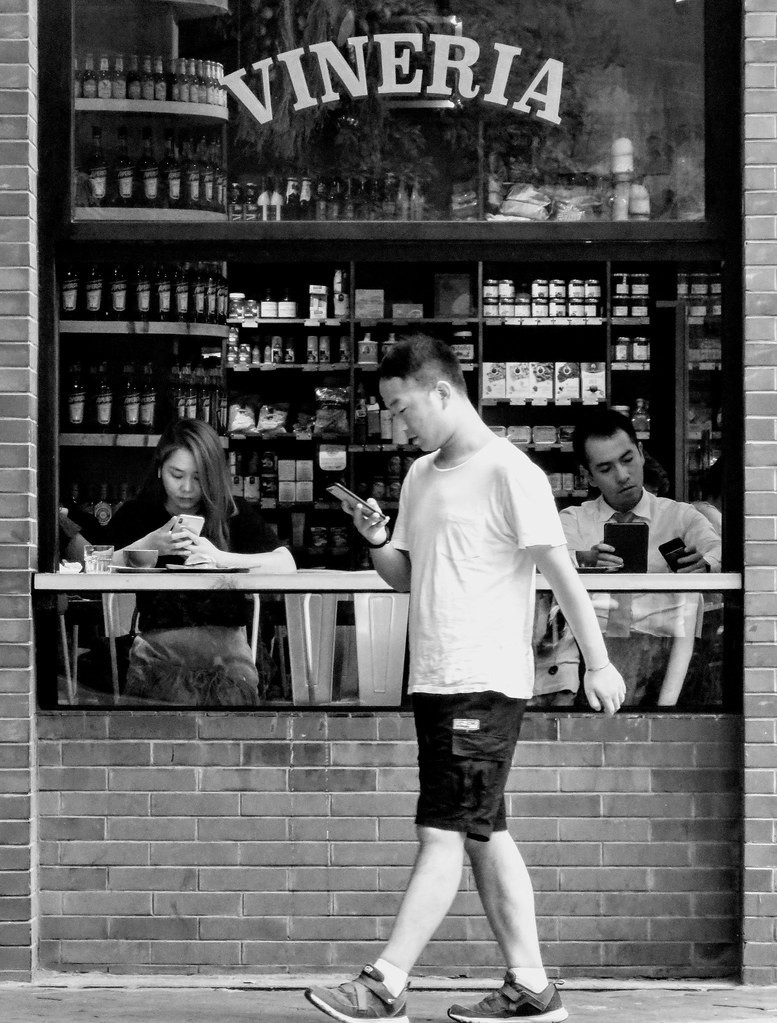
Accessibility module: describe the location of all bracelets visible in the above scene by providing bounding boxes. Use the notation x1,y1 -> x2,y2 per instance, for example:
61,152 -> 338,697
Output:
584,661 -> 611,672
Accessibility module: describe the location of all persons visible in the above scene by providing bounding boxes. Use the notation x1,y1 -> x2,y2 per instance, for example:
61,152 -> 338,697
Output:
303,337 -> 628,1023
557,408 -> 720,706
57,511 -> 94,576
100,415 -> 297,708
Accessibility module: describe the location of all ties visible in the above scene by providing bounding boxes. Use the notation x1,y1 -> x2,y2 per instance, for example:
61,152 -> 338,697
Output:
605,510 -> 638,638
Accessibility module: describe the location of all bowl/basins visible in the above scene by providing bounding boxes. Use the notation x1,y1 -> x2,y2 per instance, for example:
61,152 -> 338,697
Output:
123,550 -> 159,568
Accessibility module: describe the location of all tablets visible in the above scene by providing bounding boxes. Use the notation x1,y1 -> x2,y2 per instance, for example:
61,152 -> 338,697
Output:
603,522 -> 647,574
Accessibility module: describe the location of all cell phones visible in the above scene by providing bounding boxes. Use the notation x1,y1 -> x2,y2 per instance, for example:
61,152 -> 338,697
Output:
171,514 -> 205,541
327,483 -> 386,523
659,537 -> 695,573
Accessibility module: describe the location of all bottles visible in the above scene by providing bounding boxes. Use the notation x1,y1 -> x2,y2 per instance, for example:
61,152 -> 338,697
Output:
76,121 -> 421,218
630,397 -> 650,432
74,52 -> 228,105
59,257 -> 350,325
575,465 -> 588,490
62,338 -> 406,515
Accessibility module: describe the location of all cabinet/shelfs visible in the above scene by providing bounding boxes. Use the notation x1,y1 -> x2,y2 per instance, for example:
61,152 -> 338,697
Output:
56,0 -> 729,614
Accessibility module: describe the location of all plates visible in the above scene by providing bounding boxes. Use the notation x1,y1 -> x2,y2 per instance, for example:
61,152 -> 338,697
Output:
116,565 -> 167,573
576,566 -> 620,573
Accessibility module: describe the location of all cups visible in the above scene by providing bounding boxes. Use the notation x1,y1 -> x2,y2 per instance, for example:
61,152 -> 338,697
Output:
83,545 -> 114,574
548,472 -> 574,490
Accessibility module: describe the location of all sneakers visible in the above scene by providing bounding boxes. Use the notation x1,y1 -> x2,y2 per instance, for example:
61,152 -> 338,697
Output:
446,969 -> 569,1023
304,962 -> 410,1023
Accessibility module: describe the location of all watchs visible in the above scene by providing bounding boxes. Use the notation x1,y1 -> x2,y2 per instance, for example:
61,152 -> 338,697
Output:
365,526 -> 390,548
